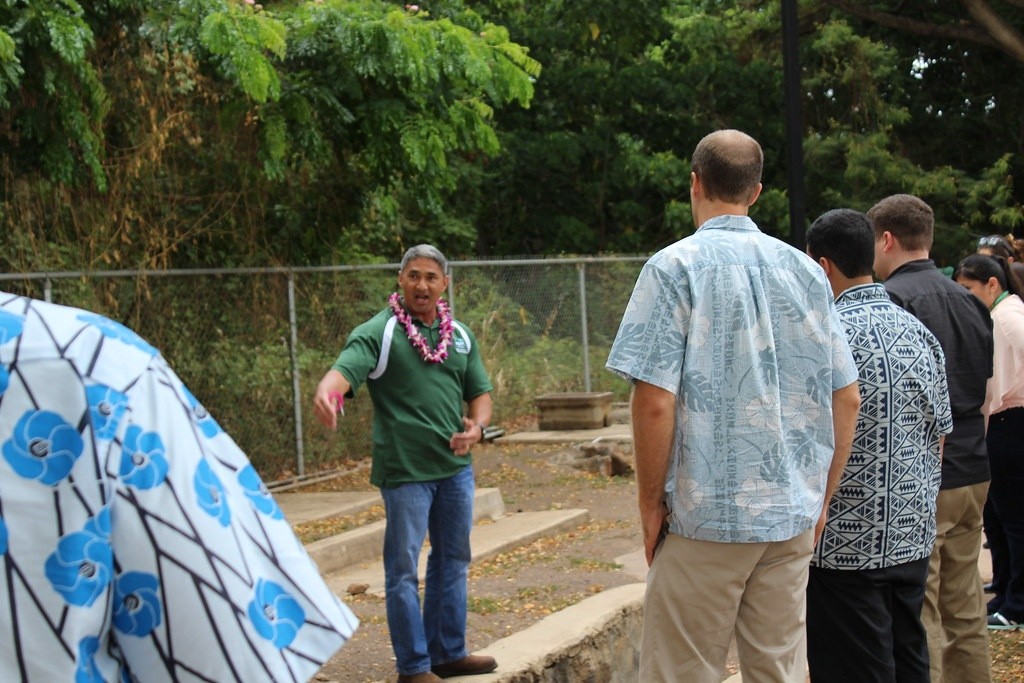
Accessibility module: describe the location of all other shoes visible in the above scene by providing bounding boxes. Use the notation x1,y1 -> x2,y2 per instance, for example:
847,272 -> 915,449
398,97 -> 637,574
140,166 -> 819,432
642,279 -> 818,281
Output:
431,652 -> 498,679
987,611 -> 1024,631
984,583 -> 995,592
397,672 -> 448,683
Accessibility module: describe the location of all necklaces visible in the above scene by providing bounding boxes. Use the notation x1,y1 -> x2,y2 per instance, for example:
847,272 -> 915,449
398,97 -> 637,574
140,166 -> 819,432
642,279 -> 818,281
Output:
389,290 -> 453,364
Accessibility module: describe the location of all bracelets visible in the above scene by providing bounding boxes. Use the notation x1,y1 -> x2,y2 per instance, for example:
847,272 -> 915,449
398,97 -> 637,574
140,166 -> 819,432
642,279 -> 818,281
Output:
479,423 -> 487,442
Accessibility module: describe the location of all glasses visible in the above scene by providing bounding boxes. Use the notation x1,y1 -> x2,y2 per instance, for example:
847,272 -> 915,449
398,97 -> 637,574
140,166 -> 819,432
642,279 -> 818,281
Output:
978,236 -> 1003,247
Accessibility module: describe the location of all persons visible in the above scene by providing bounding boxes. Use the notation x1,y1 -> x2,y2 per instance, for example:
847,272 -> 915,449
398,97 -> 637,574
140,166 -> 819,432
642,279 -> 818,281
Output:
0,292 -> 359,683
806,194 -> 1024,683
603,126 -> 863,683
311,245 -> 499,682
